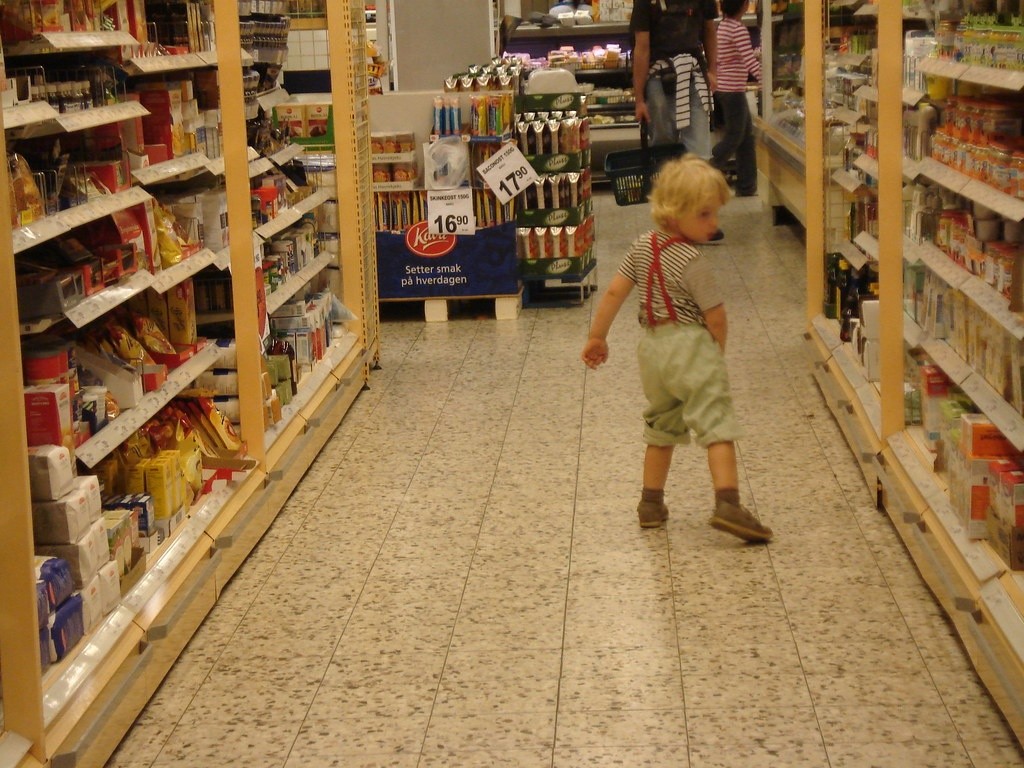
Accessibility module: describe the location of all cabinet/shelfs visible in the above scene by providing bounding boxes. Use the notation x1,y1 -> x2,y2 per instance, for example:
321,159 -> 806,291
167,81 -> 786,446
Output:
761,0 -> 1024,753
0,0 -> 382,768
491,0 -> 761,186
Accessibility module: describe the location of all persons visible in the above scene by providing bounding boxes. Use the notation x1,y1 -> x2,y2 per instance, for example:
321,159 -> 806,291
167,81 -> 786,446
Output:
709,0 -> 759,197
629,0 -> 725,246
581,154 -> 773,542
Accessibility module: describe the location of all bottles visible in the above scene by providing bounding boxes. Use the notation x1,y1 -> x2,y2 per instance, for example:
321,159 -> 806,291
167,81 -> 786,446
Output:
31,74 -> 93,115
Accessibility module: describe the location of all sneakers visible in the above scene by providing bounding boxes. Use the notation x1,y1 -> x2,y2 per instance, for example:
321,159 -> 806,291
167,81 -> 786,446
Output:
637,502 -> 669,527
710,501 -> 773,542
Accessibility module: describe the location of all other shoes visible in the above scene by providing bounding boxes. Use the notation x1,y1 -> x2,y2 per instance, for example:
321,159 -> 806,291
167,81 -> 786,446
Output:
708,229 -> 725,244
